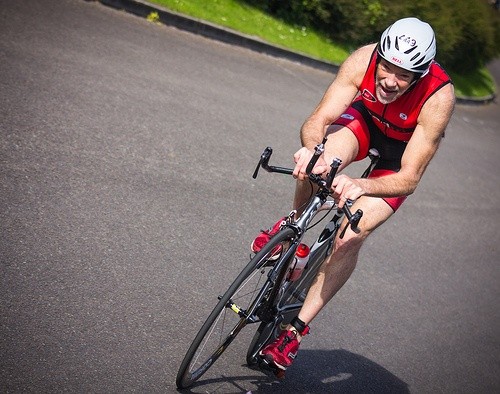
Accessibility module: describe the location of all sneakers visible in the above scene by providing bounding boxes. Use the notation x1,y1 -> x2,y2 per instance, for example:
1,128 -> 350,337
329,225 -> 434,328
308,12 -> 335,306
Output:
259,328 -> 299,370
252,216 -> 291,261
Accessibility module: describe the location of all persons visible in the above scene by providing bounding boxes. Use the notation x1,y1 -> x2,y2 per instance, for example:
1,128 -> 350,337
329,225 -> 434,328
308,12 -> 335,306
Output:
251,17 -> 455,370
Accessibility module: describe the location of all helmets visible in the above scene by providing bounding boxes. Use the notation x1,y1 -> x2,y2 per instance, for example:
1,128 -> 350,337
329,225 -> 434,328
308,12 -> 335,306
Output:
377,17 -> 436,78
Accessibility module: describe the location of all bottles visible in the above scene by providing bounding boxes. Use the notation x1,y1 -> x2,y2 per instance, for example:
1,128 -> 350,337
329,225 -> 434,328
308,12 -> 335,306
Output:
286,244 -> 310,282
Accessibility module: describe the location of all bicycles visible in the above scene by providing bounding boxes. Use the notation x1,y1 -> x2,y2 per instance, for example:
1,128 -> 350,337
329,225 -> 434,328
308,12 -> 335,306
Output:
174,132 -> 363,391
